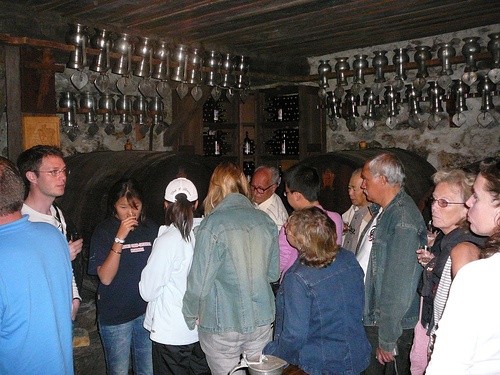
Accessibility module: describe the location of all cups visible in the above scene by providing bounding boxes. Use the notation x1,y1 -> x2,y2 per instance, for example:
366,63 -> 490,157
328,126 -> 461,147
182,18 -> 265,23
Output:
420,245 -> 432,265
127,213 -> 137,224
71,231 -> 81,243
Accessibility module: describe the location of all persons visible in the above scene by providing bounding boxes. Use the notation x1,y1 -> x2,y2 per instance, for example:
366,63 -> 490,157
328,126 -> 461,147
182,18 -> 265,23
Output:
425,157 -> 499,375
408,168 -> 480,375
87,176 -> 158,375
261,205 -> 372,374
360,151 -> 428,374
279,163 -> 345,285
138,176 -> 211,374
1,154 -> 75,375
247,164 -> 290,340
17,145 -> 83,322
341,167 -> 383,284
182,160 -> 280,374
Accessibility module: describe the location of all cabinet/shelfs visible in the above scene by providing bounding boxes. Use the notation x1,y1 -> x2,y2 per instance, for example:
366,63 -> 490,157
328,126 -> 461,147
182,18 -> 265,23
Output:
173,88 -> 243,170
257,85 -> 326,161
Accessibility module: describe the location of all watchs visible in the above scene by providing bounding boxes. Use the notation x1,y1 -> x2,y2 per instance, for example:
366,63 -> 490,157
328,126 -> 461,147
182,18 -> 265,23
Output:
114,237 -> 126,244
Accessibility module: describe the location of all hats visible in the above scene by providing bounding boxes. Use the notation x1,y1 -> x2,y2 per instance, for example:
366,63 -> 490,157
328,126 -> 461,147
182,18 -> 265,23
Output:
164,178 -> 198,202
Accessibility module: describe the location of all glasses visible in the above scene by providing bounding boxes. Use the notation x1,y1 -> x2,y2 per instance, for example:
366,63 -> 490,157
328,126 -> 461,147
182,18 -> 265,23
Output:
429,196 -> 465,206
36,167 -> 71,177
250,182 -> 275,194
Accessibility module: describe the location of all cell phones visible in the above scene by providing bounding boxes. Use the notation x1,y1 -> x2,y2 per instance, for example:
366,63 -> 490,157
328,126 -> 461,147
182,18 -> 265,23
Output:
71,232 -> 80,242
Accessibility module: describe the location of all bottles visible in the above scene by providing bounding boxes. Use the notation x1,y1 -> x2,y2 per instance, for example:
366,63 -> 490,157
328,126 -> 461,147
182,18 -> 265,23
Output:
203,94 -> 229,122
243,130 -> 255,154
265,94 -> 300,121
262,125 -> 299,156
243,162 -> 256,183
275,164 -> 285,197
203,127 -> 232,156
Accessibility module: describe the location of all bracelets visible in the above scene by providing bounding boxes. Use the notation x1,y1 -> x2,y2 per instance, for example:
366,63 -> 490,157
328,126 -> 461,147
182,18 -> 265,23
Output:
110,248 -> 121,255
